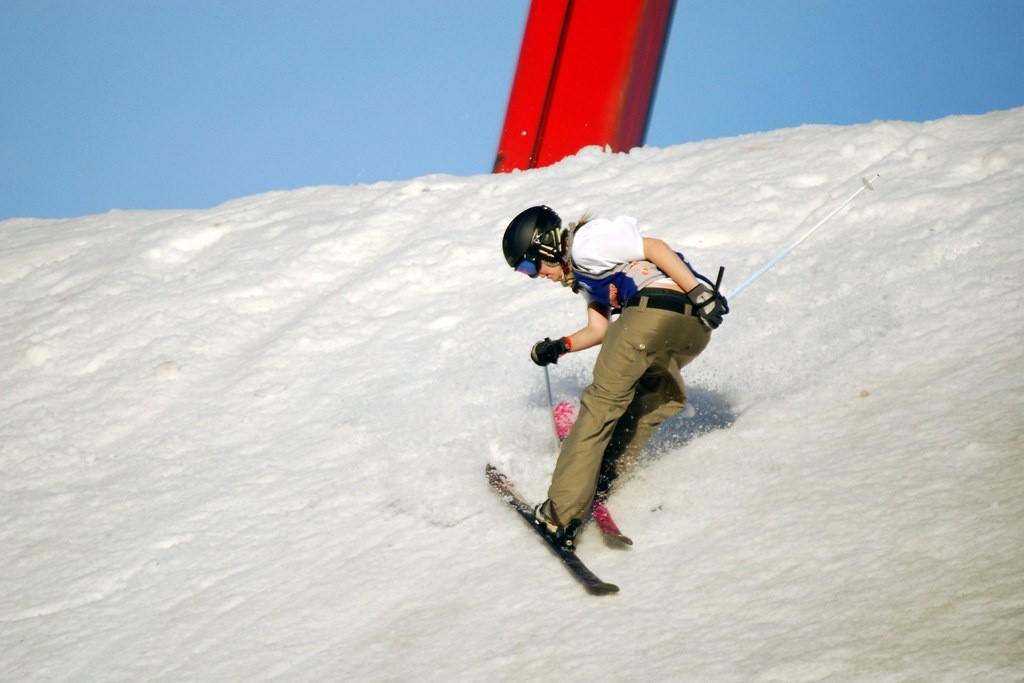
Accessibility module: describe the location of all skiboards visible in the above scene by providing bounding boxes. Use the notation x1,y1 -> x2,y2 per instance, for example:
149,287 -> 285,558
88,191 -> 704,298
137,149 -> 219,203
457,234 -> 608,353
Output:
482,398 -> 634,597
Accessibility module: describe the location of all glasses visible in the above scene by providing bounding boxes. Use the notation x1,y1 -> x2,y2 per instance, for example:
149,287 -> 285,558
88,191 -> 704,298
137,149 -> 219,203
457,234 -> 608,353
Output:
516,256 -> 539,277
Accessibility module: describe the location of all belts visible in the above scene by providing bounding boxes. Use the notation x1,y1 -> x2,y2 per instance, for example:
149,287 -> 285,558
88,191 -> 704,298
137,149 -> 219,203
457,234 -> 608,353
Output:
624,294 -> 698,316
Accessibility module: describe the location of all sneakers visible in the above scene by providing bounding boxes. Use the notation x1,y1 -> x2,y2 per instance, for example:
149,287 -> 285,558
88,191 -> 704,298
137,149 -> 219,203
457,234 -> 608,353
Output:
534,499 -> 582,552
595,476 -> 611,501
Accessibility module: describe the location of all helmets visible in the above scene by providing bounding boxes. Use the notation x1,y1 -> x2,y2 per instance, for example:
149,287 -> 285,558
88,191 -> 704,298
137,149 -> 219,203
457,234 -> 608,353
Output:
502,204 -> 562,268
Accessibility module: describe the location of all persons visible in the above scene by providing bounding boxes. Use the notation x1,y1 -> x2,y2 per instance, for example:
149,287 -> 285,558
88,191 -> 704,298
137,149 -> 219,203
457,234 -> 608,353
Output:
502,204 -> 729,555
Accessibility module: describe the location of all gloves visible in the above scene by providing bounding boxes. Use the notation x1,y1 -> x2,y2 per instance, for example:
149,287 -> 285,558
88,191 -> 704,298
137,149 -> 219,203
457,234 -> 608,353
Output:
530,336 -> 569,366
686,283 -> 729,328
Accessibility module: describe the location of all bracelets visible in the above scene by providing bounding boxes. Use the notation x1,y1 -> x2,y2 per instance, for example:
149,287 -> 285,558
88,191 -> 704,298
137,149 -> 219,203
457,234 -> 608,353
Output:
563,336 -> 573,351
